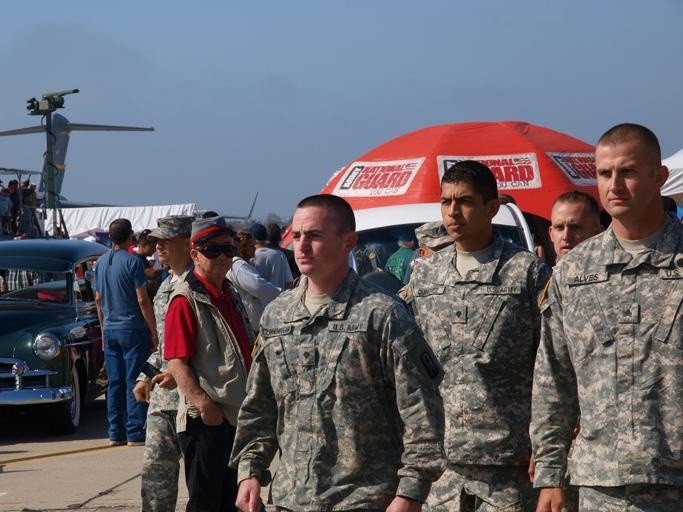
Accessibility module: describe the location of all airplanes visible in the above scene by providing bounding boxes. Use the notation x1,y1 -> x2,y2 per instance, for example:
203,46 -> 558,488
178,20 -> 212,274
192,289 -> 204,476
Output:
0,113 -> 259,220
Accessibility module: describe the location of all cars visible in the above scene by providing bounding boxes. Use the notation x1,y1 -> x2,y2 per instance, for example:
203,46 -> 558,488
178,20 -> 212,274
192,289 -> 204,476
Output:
0,238 -> 111,437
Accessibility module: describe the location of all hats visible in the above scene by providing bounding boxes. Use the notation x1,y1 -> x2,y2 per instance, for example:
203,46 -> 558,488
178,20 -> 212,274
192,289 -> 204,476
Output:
242,224 -> 265,240
189,216 -> 231,244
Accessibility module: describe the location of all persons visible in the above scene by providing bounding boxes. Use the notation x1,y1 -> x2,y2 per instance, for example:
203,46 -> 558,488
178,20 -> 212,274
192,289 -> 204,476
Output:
165,216 -> 255,512
3,180 -> 35,235
133,218 -> 193,512
76,195 -> 683,444
532,123 -> 683,512
396,160 -> 551,511
228,194 -> 447,512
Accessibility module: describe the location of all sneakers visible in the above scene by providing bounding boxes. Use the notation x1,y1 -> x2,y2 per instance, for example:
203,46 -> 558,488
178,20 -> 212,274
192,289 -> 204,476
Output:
148,217 -> 192,239
110,440 -> 145,447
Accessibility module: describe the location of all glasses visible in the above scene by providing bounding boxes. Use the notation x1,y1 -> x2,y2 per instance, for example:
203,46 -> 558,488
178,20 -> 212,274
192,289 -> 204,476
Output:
198,243 -> 238,260
